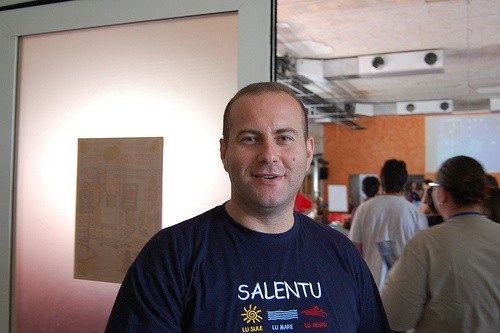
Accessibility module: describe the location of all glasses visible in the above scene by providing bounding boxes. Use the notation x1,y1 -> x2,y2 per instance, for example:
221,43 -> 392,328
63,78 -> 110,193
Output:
428,181 -> 440,189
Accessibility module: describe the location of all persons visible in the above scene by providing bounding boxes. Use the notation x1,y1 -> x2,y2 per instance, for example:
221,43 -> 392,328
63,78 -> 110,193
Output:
104,82 -> 390,333
379,155 -> 500,333
295,159 -> 500,333
